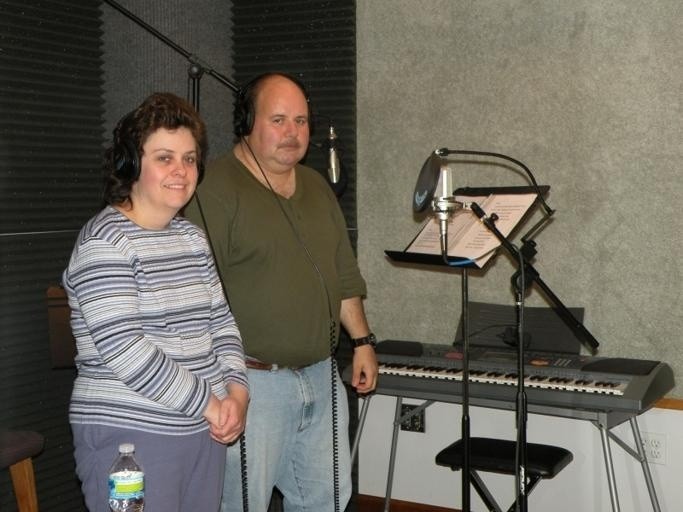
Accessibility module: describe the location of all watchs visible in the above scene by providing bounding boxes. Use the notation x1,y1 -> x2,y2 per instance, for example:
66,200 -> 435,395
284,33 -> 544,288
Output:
348,333 -> 377,349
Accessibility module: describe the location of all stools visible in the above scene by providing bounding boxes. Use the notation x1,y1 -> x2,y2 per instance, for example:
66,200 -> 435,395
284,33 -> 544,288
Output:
435,438 -> 572,512
0,429 -> 45,512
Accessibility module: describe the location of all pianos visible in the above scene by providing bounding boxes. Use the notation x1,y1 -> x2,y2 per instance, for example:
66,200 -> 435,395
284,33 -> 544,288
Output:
341,340 -> 673,415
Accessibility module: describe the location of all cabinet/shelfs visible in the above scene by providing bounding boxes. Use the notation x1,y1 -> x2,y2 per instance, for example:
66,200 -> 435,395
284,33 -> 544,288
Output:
349,387 -> 661,512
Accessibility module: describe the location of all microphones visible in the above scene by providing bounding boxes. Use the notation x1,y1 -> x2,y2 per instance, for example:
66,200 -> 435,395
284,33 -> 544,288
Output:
325,124 -> 342,184
413,147 -> 553,215
429,166 -> 463,254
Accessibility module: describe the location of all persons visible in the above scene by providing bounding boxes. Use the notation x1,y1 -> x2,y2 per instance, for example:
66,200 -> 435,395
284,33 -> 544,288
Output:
61,91 -> 250,512
185,72 -> 380,512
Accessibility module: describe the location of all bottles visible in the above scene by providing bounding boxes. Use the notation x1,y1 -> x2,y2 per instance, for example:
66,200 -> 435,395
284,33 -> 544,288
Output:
104,443 -> 147,512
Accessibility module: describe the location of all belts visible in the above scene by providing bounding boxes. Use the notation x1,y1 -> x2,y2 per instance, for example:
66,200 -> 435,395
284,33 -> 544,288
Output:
244,360 -> 274,371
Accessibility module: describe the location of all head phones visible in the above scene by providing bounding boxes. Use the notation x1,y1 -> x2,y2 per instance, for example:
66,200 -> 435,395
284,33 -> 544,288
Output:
113,110 -> 205,186
238,73 -> 315,139
